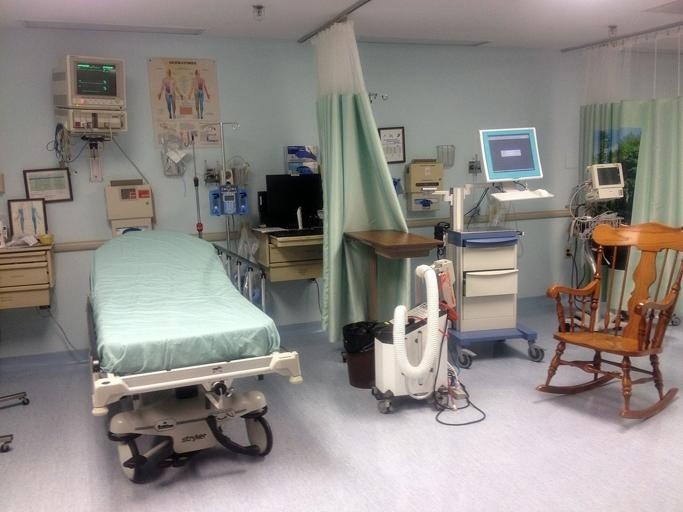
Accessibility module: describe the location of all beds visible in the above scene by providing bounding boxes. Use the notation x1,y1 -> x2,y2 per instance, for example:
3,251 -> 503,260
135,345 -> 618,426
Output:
87,227 -> 306,486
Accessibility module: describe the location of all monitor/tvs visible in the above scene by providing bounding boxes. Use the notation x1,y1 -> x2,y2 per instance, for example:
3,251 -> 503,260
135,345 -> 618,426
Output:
591,163 -> 624,188
266,174 -> 323,227
479,127 -> 543,183
53,54 -> 127,111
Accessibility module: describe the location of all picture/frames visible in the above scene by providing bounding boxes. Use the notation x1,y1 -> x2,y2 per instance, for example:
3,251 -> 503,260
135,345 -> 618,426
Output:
7,197 -> 48,239
20,166 -> 73,203
377,126 -> 405,164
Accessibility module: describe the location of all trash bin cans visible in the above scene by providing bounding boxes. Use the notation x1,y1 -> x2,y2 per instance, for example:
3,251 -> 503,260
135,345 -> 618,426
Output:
342,321 -> 377,389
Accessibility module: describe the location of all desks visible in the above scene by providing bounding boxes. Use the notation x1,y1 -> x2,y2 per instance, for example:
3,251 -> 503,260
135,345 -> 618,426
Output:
341,228 -> 445,322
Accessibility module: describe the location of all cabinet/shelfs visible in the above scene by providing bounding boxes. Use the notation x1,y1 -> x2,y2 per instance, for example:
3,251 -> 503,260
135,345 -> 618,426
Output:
446,224 -> 524,334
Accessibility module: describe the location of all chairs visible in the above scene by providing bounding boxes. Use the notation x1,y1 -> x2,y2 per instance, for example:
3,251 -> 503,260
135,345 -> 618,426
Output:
535,219 -> 682,419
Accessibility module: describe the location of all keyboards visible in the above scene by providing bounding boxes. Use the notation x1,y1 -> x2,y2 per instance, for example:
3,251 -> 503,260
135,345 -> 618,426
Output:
272,228 -> 322,237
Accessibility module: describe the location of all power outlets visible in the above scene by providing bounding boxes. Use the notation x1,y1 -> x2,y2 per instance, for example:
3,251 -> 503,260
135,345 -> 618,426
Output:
309,277 -> 321,284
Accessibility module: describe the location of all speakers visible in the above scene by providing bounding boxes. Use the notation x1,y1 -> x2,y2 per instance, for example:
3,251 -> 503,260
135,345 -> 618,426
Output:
258,191 -> 266,228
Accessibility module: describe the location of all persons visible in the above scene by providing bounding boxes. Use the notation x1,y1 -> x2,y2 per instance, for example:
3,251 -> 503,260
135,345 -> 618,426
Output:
188,70 -> 211,119
157,69 -> 183,119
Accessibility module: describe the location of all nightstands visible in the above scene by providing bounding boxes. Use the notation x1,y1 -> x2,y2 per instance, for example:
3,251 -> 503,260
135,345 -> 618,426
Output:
248,228 -> 326,285
0,241 -> 54,310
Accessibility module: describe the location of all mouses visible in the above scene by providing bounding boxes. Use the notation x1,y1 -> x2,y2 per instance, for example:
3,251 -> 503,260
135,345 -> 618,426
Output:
535,189 -> 549,197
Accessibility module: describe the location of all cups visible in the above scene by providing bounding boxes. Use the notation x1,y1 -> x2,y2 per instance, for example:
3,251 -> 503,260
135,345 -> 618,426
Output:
38,234 -> 53,245
